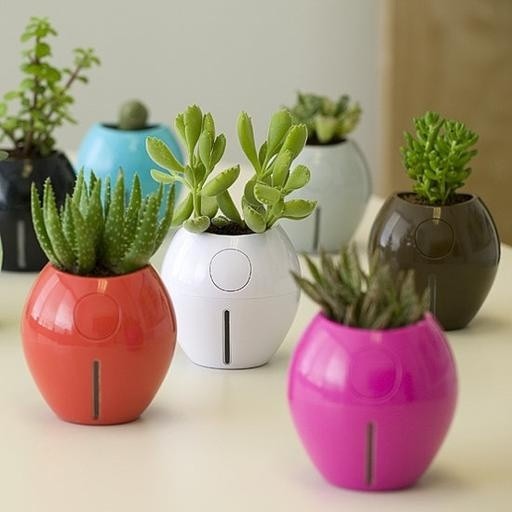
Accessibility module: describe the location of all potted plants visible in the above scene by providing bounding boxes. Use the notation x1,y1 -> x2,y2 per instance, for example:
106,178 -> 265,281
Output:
0,20 -> 99,273
283,247 -> 463,491
22,165 -> 179,430
266,92 -> 374,258
368,111 -> 501,335
144,104 -> 315,374
76,99 -> 186,222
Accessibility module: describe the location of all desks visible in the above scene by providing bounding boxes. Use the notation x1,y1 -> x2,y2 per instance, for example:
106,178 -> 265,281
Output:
1,193 -> 512,512
